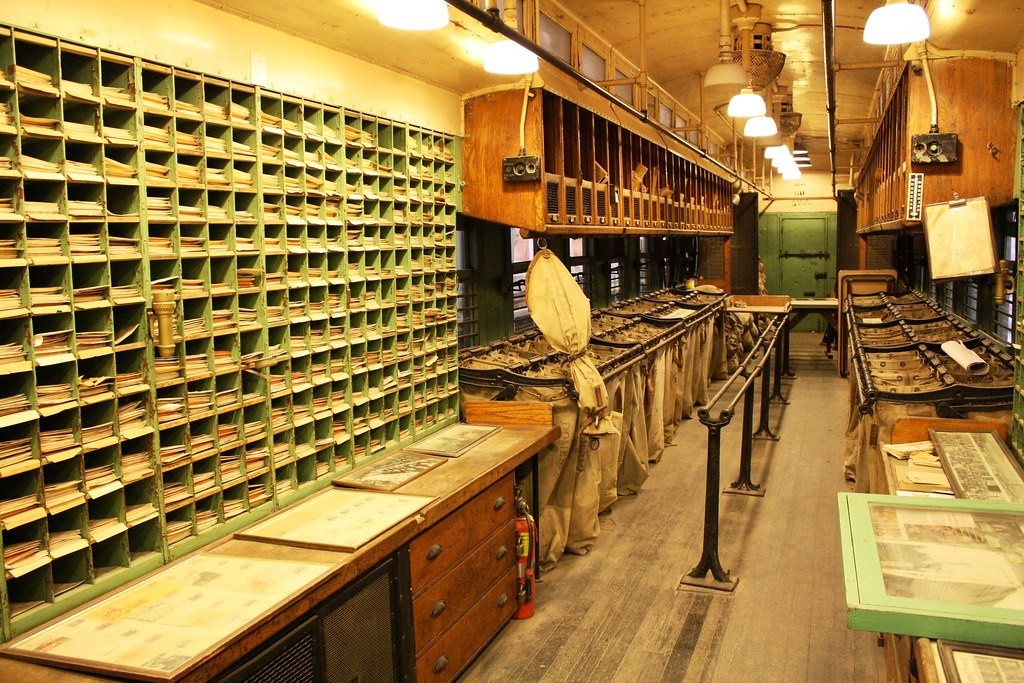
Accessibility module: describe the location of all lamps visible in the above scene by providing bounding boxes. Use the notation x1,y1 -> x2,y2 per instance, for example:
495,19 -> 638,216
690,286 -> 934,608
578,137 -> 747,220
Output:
727,88 -> 813,180
732,188 -> 743,206
863,0 -> 931,46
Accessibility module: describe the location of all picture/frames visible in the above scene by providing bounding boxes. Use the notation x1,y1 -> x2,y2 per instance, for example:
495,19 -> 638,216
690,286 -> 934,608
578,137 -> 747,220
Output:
403,423 -> 504,458
332,450 -> 449,491
233,486 -> 441,553
937,639 -> 1024,683
927,427 -> 1024,502
0,551 -> 348,683
837,492 -> 1024,651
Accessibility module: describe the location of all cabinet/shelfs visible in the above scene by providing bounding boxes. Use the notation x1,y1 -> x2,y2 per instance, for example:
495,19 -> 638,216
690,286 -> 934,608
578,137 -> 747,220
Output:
1007,99 -> 1024,468
174,468 -> 520,683
855,58 -> 1017,237
0,20 -> 464,645
461,86 -> 736,240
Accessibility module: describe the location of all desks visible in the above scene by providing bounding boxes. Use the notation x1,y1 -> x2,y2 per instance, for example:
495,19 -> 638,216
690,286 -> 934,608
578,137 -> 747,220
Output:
723,294 -> 838,367
